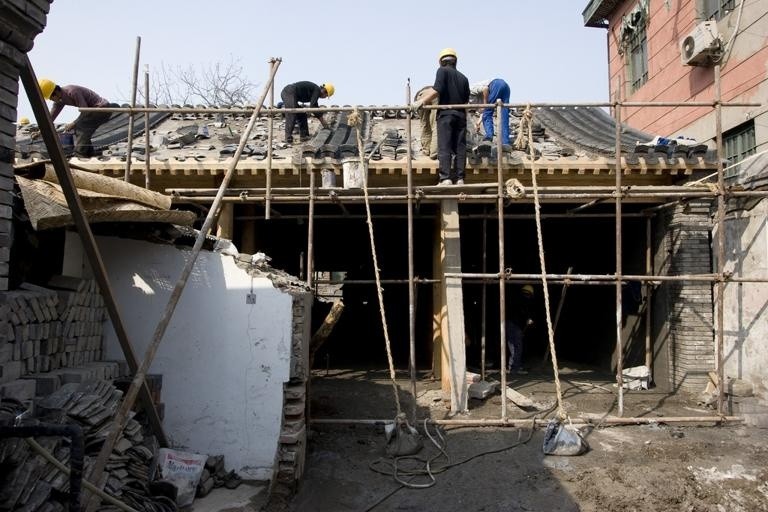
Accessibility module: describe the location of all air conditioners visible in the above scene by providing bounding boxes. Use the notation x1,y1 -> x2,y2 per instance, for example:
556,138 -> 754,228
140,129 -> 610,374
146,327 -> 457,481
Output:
680,20 -> 722,65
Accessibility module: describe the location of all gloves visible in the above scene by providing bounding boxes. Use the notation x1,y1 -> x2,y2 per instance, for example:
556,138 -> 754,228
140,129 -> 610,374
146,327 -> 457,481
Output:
410,100 -> 423,113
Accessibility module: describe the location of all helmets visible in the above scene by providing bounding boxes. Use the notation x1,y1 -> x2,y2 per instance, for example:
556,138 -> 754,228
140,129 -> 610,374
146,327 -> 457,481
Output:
20,117 -> 29,124
522,284 -> 534,295
38,79 -> 56,100
321,83 -> 334,99
439,48 -> 457,62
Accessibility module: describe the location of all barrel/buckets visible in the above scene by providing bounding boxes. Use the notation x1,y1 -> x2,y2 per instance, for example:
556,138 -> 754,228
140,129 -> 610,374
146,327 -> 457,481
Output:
342,156 -> 370,189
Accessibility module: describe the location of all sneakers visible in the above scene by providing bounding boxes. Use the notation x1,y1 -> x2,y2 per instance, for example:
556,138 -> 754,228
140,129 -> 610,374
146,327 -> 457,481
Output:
422,149 -> 437,159
287,136 -> 311,142
436,179 -> 465,186
511,367 -> 528,375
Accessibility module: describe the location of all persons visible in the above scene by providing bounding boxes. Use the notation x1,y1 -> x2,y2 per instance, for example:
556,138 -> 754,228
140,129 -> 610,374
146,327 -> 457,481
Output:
468,78 -> 513,153
281,81 -> 334,146
505,284 -> 536,375
420,49 -> 469,193
414,86 -> 440,161
30,78 -> 111,174
